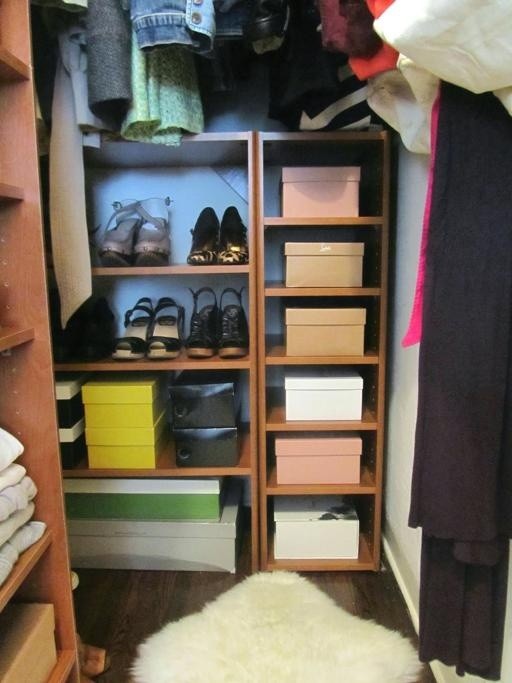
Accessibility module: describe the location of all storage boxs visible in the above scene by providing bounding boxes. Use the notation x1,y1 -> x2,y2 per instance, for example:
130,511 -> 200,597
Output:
0,603 -> 59,683
282,239 -> 366,290
283,306 -> 368,358
269,495 -> 363,563
58,417 -> 86,470
272,430 -> 365,487
84,401 -> 174,469
282,369 -> 365,423
169,369 -> 243,431
64,478 -> 232,522
54,380 -> 83,429
62,482 -> 243,576
68,371 -> 182,428
173,428 -> 243,470
279,164 -> 363,218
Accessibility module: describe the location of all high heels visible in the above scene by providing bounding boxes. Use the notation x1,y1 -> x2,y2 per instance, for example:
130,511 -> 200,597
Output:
97,199 -> 146,266
217,288 -> 249,358
187,287 -> 220,358
111,296 -> 156,361
147,295 -> 186,360
133,195 -> 172,264
218,204 -> 250,265
188,206 -> 221,266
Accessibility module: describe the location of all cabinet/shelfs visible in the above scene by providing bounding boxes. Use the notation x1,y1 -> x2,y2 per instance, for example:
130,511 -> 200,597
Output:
0,1 -> 85,682
38,131 -> 256,576
257,128 -> 391,574
